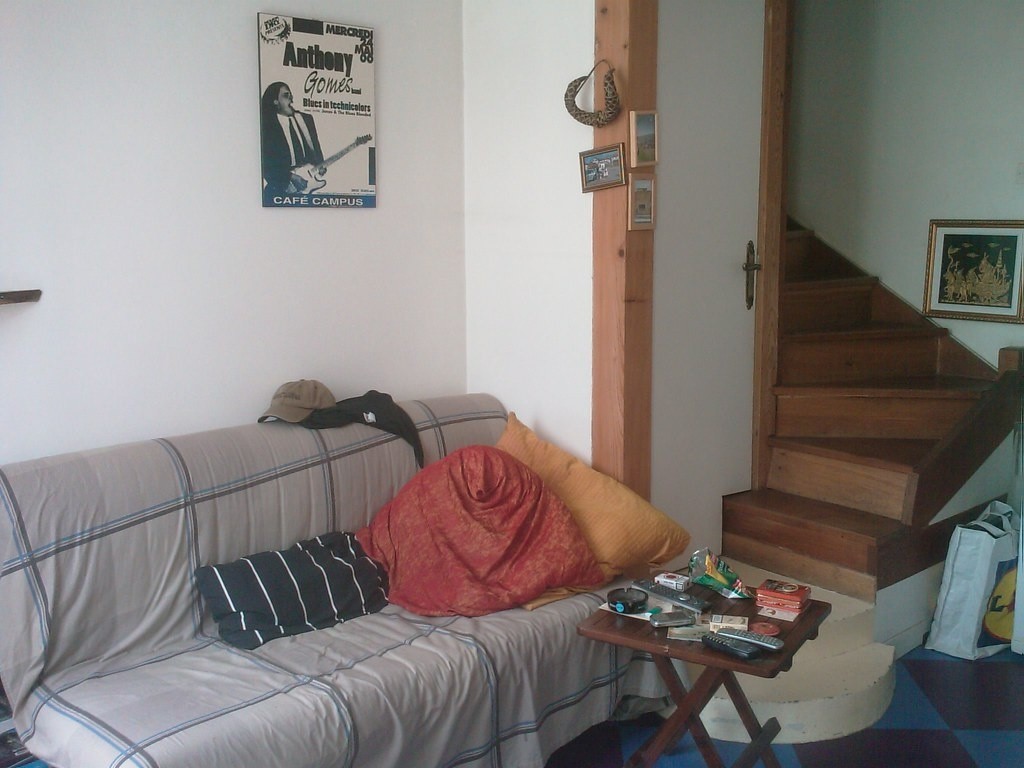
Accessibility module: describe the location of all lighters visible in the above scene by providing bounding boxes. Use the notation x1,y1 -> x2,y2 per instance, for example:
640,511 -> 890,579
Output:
643,605 -> 663,618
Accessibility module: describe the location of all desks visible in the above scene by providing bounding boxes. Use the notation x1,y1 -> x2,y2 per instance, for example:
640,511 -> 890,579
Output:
577,569 -> 832,768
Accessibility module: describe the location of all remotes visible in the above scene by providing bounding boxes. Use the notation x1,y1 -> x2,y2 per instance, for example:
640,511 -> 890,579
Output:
702,634 -> 761,660
631,579 -> 712,613
716,628 -> 785,651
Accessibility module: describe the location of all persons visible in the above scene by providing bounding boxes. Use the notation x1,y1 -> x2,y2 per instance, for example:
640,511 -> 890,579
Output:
261,81 -> 327,195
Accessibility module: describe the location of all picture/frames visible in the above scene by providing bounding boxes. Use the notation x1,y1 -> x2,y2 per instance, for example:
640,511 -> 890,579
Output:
629,110 -> 658,168
627,173 -> 656,231
923,219 -> 1024,325
579,142 -> 627,193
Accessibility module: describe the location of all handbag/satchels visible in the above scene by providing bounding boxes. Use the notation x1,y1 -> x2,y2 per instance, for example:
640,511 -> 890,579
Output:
925,501 -> 1021,661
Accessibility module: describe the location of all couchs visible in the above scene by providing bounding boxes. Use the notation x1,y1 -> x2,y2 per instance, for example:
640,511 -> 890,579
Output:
0,393 -> 692,768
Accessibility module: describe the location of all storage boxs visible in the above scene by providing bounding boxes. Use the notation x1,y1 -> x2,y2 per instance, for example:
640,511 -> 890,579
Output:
710,615 -> 749,632
655,572 -> 693,592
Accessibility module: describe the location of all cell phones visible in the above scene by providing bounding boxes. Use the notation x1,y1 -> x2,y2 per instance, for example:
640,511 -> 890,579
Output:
649,611 -> 696,627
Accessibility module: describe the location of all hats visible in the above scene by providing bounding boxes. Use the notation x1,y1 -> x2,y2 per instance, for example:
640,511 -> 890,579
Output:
257,380 -> 337,426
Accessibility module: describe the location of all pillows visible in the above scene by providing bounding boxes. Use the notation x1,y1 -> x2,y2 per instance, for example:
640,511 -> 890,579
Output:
354,446 -> 607,618
496,412 -> 691,612
194,532 -> 389,650
